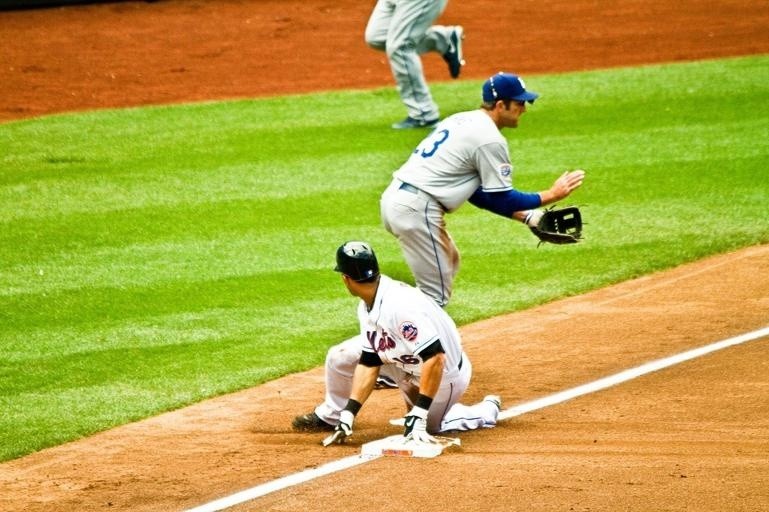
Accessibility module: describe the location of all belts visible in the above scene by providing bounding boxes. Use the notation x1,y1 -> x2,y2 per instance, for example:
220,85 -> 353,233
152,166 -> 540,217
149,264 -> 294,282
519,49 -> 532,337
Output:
400,182 -> 447,211
458,353 -> 463,370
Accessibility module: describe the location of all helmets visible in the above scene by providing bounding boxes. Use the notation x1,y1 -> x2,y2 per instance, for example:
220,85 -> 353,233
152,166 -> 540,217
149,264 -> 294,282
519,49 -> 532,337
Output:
334,239 -> 380,280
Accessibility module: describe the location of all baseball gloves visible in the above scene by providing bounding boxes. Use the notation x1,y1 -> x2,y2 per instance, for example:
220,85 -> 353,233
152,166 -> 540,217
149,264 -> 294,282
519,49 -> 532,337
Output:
523,204 -> 588,249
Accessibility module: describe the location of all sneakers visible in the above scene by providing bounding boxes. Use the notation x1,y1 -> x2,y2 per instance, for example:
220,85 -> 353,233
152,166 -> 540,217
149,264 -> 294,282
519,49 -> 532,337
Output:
373,375 -> 399,389
443,24 -> 465,78
391,117 -> 440,128
483,393 -> 501,410
291,411 -> 336,432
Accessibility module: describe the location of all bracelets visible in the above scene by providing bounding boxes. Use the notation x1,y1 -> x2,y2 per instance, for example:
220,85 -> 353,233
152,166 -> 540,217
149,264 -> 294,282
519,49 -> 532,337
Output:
413,393 -> 434,410
522,210 -> 536,226
342,398 -> 363,417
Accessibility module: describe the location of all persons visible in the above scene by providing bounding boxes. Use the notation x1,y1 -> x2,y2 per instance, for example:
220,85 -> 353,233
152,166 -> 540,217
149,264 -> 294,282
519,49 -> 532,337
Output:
290,239 -> 501,449
378,70 -> 586,308
364,1 -> 467,131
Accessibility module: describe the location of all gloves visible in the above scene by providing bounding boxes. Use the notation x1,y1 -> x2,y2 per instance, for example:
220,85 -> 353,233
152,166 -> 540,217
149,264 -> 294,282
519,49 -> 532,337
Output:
389,405 -> 440,446
321,410 -> 355,447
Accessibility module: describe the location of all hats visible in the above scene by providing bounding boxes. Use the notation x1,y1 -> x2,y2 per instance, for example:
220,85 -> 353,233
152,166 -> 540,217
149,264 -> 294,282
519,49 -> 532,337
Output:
483,72 -> 538,102
